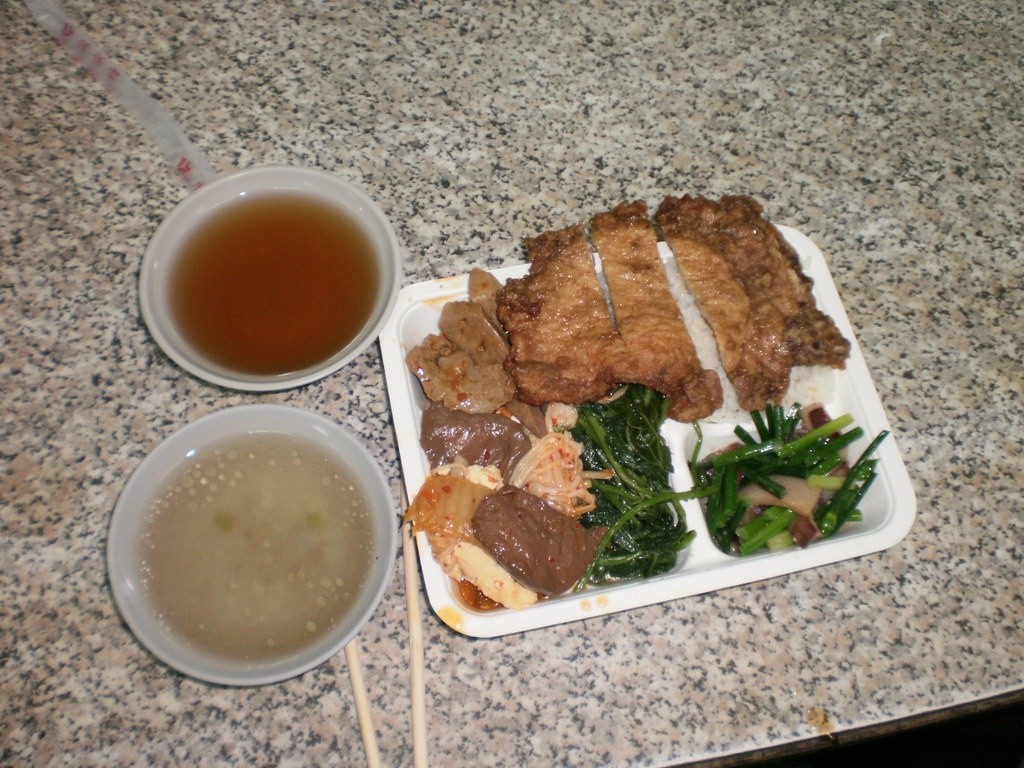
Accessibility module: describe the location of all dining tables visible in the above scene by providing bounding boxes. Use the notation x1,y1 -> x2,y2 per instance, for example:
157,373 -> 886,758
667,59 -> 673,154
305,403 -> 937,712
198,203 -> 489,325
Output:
2,0 -> 1024,768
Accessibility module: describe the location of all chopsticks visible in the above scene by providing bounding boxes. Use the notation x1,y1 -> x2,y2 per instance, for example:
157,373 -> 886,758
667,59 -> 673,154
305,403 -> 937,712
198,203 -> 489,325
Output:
339,481 -> 432,767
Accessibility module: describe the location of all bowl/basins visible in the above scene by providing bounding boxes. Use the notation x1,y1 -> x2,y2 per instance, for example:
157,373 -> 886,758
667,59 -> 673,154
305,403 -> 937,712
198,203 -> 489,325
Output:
106,402 -> 401,687
137,163 -> 402,393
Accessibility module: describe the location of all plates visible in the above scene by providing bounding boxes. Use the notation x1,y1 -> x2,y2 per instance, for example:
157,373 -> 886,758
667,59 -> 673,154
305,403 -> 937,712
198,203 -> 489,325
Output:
376,223 -> 917,640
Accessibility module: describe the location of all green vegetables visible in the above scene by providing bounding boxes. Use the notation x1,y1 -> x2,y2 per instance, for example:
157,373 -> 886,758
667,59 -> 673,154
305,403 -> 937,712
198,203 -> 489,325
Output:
572,385 -> 890,585
